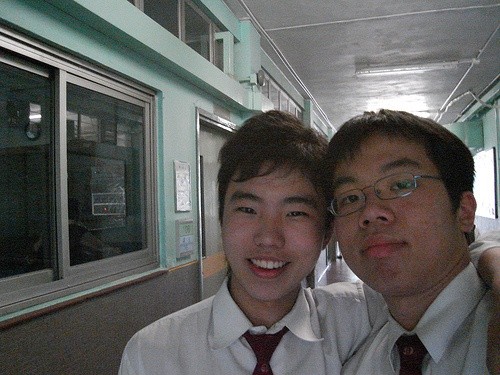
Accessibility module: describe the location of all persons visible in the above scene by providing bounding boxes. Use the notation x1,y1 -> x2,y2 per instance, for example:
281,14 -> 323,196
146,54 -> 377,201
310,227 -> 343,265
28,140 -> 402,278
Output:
325,110 -> 500,375
118,110 -> 500,375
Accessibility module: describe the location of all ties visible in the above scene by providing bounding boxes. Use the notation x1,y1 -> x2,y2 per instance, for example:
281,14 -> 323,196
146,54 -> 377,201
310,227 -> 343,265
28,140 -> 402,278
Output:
394,332 -> 428,374
240,326 -> 293,374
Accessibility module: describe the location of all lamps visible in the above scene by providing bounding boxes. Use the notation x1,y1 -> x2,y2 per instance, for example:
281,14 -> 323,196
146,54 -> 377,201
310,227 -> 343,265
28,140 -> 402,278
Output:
355,60 -> 459,75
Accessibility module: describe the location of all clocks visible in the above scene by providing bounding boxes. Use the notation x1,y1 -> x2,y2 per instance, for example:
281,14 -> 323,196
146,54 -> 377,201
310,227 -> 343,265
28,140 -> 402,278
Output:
24,122 -> 42,140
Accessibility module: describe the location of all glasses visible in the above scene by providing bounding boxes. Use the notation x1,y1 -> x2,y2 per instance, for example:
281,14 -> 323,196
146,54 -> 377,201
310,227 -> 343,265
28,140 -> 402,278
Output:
325,170 -> 450,217
